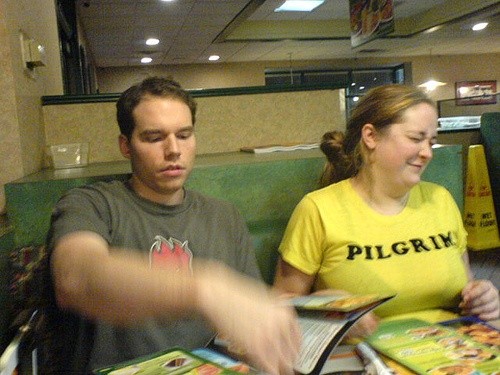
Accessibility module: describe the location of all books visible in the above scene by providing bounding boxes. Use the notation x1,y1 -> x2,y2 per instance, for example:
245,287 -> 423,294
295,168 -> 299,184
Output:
240,142 -> 321,153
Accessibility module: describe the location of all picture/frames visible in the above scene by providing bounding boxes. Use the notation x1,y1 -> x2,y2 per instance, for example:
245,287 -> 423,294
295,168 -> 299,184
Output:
455,80 -> 498,105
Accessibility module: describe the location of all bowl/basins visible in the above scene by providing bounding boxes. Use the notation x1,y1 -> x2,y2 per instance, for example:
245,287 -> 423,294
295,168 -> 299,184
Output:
44,141 -> 91,169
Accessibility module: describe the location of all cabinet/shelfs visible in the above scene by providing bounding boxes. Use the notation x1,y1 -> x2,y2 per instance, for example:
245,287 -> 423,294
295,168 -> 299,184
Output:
5,144 -> 466,284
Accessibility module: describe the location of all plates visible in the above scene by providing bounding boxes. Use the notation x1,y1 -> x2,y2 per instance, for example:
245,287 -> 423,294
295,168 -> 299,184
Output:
352,8 -> 393,39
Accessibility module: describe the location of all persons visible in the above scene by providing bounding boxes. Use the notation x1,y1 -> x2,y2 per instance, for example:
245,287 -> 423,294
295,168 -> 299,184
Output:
277,84 -> 500,344
46,77 -> 300,375
